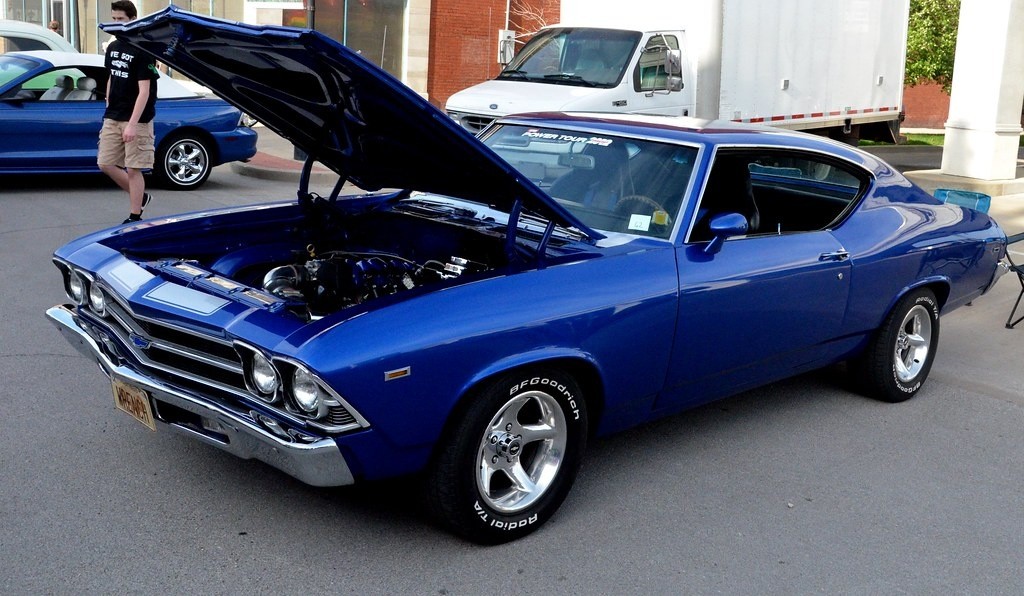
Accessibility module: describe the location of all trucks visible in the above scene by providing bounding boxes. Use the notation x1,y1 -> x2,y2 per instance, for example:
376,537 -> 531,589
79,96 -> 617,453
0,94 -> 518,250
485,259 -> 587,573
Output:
443,0 -> 913,179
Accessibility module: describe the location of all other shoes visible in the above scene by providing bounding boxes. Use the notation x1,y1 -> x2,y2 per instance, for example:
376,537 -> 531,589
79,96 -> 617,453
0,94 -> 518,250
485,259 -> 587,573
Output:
139,192 -> 153,216
122,218 -> 142,224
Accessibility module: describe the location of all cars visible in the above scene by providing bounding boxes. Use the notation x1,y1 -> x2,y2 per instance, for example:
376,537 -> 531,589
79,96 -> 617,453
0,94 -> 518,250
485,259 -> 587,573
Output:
0,20 -> 86,87
43,6 -> 1014,544
1,48 -> 262,191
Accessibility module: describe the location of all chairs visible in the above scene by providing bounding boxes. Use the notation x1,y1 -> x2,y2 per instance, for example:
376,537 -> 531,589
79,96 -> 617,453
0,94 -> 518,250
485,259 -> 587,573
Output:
691,156 -> 762,237
40,74 -> 97,100
548,138 -> 635,216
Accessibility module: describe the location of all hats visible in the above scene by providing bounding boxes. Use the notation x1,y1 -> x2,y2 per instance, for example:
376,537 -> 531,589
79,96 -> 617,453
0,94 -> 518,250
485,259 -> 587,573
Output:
49,21 -> 59,28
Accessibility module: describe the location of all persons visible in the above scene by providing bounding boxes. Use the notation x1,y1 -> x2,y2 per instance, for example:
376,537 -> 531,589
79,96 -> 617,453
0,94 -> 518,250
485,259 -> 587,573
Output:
99,1 -> 163,224
